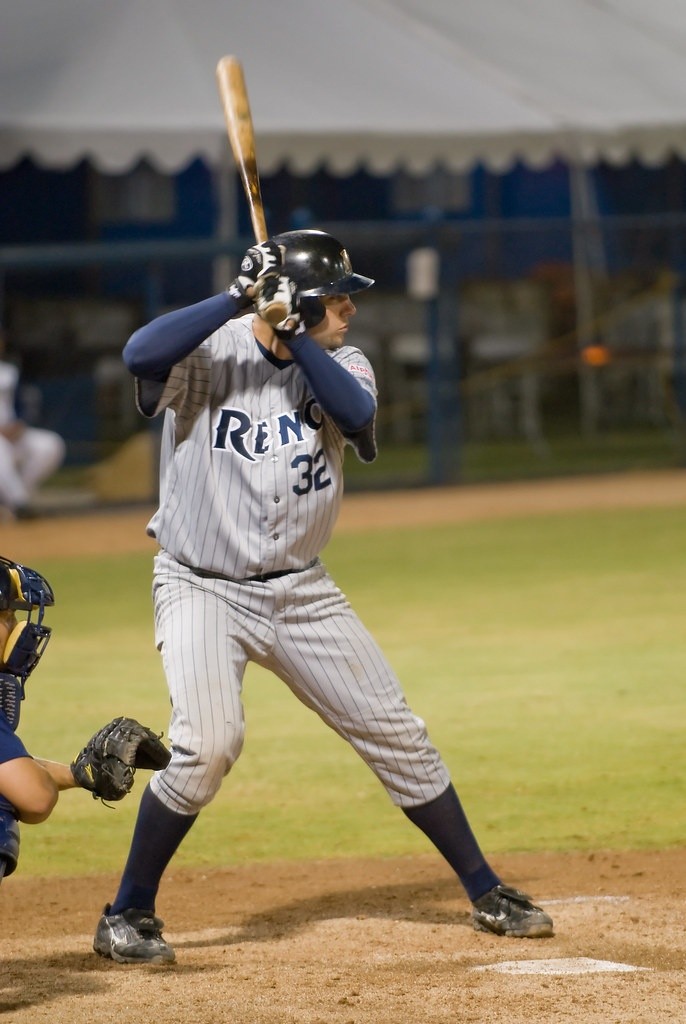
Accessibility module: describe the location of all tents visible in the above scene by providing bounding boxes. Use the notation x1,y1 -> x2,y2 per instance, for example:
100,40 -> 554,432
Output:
0,0 -> 686,440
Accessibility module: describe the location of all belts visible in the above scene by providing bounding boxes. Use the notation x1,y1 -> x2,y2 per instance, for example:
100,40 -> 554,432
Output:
175,556 -> 320,583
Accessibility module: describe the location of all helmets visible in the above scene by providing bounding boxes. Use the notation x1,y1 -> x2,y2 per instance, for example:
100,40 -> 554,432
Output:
0,556 -> 56,611
273,230 -> 374,329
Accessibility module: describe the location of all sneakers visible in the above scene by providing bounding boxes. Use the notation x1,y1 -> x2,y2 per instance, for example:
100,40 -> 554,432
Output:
93,902 -> 176,964
471,882 -> 556,938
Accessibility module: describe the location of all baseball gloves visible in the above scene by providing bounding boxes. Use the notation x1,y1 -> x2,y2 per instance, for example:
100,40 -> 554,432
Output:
70,714 -> 171,802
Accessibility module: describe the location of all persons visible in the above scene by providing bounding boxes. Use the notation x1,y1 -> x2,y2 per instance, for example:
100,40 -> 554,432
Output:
0,357 -> 64,523
89,232 -> 552,967
0,557 -> 170,882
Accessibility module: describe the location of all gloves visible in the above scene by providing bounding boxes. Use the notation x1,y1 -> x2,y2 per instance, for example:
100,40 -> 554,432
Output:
227,240 -> 282,310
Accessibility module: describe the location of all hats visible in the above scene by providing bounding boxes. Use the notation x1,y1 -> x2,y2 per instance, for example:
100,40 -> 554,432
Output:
251,272 -> 308,341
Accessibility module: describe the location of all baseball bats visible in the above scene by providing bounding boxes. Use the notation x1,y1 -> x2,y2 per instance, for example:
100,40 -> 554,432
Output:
211,50 -> 292,327
